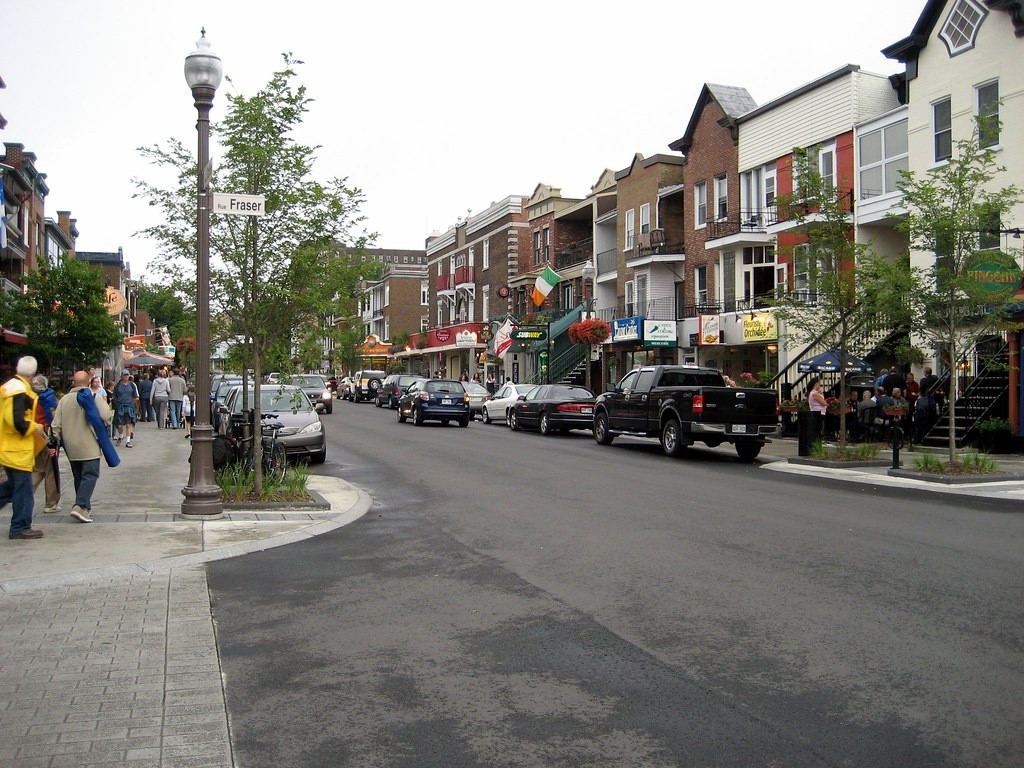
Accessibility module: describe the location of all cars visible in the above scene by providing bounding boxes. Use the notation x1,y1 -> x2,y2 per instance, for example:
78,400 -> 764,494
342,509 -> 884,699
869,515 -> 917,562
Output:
210,371 -> 334,464
336,376 -> 355,400
454,381 -> 493,422
509,384 -> 599,435
481,382 -> 538,427
396,379 -> 470,428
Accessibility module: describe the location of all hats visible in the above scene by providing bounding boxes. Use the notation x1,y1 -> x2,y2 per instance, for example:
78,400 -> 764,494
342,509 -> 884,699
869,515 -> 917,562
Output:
121,369 -> 130,375
881,369 -> 887,375
889,367 -> 896,372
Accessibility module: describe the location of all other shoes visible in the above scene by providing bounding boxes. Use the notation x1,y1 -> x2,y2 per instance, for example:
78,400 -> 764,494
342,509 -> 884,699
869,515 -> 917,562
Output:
185,434 -> 190,438
44,505 -> 60,513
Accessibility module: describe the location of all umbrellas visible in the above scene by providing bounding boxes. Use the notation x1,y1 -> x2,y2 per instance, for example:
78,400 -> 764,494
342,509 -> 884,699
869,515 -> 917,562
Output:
797,347 -> 875,397
121,348 -> 177,380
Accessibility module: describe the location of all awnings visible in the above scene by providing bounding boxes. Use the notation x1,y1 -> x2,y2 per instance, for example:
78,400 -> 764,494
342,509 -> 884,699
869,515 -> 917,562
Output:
0,324 -> 28,344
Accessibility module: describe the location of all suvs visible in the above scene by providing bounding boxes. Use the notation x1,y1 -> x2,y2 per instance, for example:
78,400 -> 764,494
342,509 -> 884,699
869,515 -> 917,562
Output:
375,374 -> 425,409
349,370 -> 389,403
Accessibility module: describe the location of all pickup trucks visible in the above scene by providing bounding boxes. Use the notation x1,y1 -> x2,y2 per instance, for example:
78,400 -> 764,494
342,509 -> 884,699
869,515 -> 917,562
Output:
592,365 -> 783,461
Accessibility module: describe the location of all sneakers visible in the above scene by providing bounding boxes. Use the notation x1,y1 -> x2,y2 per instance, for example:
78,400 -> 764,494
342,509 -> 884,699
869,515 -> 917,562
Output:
126,442 -> 133,448
116,436 -> 124,446
71,504 -> 93,522
9,529 -> 44,538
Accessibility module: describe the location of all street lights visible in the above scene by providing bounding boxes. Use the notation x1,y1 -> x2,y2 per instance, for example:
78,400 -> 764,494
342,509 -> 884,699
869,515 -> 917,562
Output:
580,259 -> 596,393
178,24 -> 223,516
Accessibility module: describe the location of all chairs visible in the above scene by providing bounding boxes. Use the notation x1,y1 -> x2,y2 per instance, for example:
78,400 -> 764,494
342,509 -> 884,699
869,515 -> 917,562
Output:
860,408 -> 880,443
742,215 -> 761,233
650,230 -> 666,252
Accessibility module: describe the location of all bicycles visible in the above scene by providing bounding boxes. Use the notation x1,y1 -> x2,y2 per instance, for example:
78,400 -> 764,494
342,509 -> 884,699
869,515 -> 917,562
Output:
232,408 -> 288,484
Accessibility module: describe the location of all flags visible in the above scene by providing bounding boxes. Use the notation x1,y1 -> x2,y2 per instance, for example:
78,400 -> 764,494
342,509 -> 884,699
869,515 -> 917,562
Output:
530,265 -> 562,307
0,175 -> 7,254
494,318 -> 521,359
159,326 -> 171,344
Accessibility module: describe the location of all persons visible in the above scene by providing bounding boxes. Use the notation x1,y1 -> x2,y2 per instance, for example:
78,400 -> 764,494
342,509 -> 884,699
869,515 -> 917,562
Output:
486,374 -> 496,396
807,366 -> 944,453
723,376 -> 742,388
104,368 -> 196,448
0,356 -> 115,539
420,363 -> 480,383
502,377 -> 515,388
